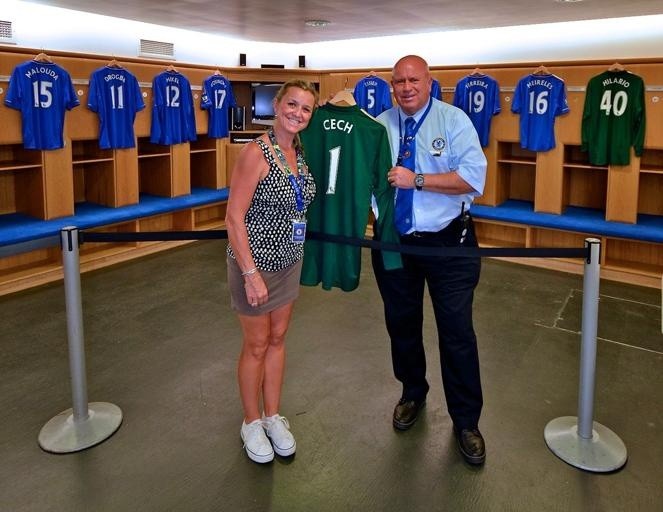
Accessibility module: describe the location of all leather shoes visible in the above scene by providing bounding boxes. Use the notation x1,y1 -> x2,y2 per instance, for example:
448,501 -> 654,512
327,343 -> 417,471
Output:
392,397 -> 426,430
454,424 -> 486,464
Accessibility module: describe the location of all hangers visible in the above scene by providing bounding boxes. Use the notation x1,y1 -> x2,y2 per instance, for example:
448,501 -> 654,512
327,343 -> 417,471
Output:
365,63 -> 637,79
328,75 -> 356,107
33,47 -> 225,75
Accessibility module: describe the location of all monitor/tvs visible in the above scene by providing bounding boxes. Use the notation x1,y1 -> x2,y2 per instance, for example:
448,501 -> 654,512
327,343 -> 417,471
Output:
251,84 -> 283,126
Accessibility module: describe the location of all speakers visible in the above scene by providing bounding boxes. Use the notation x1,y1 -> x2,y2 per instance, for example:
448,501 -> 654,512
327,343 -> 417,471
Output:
240,53 -> 246,66
231,105 -> 246,131
298,55 -> 305,68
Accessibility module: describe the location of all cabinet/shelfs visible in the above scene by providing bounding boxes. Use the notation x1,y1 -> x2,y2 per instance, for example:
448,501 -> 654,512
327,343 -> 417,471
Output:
0,51 -> 228,297
327,62 -> 661,278
225,68 -> 327,188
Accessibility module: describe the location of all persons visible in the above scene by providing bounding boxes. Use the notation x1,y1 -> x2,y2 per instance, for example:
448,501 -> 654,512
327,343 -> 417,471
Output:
314,53 -> 488,468
223,79 -> 318,465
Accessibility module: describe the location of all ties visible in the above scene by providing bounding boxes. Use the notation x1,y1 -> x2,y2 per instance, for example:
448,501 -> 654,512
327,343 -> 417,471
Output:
394,119 -> 416,234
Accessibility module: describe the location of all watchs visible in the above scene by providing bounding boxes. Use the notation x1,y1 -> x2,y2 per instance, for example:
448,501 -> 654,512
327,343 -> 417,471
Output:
414,173 -> 425,191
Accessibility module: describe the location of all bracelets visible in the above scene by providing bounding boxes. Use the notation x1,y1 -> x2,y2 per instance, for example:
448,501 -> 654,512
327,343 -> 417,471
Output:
240,267 -> 258,277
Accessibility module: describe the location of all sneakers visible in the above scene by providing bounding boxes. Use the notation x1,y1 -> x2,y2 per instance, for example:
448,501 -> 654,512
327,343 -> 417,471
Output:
240,418 -> 275,464
261,411 -> 296,456
